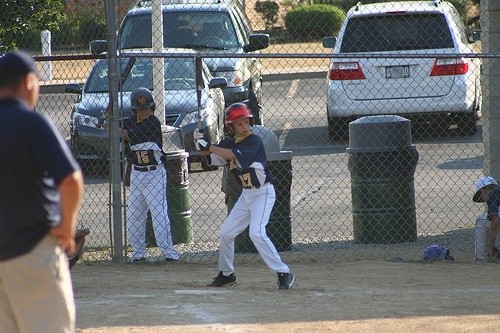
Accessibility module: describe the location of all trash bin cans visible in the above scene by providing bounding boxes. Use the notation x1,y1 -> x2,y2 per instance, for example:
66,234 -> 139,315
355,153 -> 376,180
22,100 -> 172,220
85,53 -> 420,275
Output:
345,115 -> 419,245
145,125 -> 194,247
222,126 -> 293,252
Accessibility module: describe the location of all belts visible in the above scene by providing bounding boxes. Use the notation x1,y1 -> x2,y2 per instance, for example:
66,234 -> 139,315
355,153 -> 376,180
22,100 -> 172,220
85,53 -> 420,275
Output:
134,164 -> 156,172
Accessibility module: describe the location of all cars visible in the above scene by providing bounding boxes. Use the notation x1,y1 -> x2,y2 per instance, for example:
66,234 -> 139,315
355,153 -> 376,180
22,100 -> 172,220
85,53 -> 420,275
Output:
63,46 -> 228,173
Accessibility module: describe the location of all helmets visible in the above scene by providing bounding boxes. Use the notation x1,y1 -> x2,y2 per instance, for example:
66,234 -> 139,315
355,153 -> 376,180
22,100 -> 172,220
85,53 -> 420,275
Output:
130,87 -> 156,112
224,103 -> 255,134
471,176 -> 498,203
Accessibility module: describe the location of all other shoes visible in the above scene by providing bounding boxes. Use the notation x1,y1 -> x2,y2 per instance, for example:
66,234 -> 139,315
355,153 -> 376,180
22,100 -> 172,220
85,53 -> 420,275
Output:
133,257 -> 148,263
166,257 -> 179,262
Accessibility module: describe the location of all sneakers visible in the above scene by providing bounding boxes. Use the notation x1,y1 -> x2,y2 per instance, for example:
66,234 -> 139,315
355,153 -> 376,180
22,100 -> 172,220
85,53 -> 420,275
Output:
276,273 -> 296,291
207,272 -> 237,288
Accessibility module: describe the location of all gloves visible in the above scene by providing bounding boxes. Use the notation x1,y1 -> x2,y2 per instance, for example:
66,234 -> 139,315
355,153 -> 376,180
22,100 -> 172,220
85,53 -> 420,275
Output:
99,110 -> 110,126
193,128 -> 205,141
195,138 -> 211,152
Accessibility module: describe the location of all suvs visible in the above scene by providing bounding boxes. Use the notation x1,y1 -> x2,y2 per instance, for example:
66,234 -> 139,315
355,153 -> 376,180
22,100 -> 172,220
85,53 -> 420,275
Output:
322,0 -> 482,138
88,0 -> 270,126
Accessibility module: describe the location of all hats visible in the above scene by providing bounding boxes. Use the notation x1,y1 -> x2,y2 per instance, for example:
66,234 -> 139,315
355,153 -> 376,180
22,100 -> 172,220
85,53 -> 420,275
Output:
0,50 -> 44,88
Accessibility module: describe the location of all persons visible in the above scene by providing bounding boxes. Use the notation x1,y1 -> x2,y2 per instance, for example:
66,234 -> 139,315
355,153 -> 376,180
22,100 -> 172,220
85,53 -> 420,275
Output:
473,176 -> 500,259
0,50 -> 84,333
95,87 -> 179,262
194,103 -> 295,290
